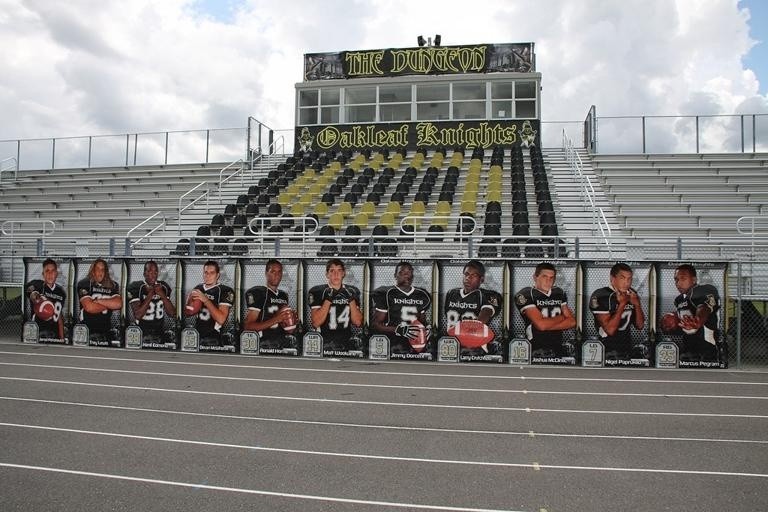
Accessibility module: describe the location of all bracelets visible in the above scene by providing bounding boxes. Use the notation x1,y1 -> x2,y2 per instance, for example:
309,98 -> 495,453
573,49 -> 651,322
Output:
204,299 -> 211,306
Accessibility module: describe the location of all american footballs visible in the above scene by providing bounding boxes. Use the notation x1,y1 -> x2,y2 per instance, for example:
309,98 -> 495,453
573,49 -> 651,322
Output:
408,320 -> 427,352
34,300 -> 54,321
281,306 -> 297,333
184,297 -> 203,318
662,312 -> 680,333
448,320 -> 496,349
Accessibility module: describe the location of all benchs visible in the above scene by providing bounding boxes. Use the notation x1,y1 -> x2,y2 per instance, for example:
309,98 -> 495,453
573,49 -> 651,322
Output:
590,154 -> 767,261
1,161 -> 243,254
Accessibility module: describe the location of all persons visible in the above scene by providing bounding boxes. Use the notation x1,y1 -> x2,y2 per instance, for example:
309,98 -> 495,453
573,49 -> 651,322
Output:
306,257 -> 364,352
588,263 -> 646,362
371,260 -> 432,357
240,259 -> 301,355
672,264 -> 723,363
25,257 -> 67,342
74,258 -> 124,343
512,262 -> 577,358
443,260 -> 503,358
183,259 -> 234,349
125,261 -> 177,346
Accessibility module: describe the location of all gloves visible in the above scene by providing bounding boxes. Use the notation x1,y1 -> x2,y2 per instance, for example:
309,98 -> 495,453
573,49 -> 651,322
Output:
394,324 -> 421,342
322,287 -> 337,302
338,285 -> 355,301
